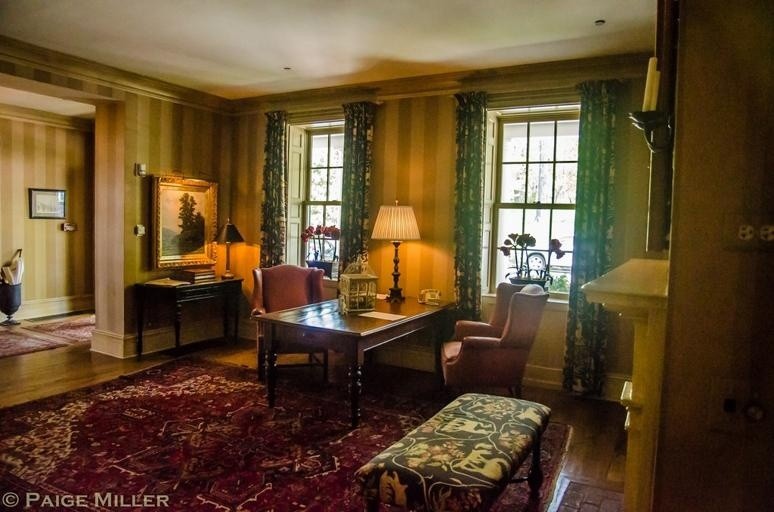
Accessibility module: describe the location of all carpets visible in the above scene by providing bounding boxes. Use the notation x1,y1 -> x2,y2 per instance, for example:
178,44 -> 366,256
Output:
0,314 -> 95,359
0,352 -> 571,510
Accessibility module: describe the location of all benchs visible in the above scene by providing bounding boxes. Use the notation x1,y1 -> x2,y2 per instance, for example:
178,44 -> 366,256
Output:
353,392 -> 551,510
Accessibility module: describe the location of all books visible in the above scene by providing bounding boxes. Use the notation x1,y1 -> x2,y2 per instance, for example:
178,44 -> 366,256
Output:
175,269 -> 216,283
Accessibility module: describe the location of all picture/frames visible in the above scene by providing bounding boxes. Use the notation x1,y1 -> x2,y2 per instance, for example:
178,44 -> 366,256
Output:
151,173 -> 219,270
28,187 -> 66,219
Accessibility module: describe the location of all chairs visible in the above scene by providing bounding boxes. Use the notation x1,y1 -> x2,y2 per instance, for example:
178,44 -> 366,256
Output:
249,263 -> 328,400
441,282 -> 548,399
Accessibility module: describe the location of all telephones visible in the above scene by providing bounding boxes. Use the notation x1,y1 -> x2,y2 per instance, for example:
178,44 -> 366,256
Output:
421,289 -> 441,306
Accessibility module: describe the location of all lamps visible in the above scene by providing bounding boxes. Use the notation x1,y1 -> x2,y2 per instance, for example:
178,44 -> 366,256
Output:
371,197 -> 420,302
213,218 -> 244,280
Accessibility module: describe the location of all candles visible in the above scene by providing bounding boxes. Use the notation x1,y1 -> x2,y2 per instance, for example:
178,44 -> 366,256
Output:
640,55 -> 663,112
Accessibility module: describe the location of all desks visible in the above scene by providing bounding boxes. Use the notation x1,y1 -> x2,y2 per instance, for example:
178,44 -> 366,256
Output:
252,293 -> 456,426
134,277 -> 243,354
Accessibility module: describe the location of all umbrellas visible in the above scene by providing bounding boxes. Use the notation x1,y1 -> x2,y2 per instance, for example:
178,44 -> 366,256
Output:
14,248 -> 24,284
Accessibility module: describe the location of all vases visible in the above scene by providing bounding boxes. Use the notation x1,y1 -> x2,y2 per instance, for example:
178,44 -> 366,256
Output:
306,260 -> 336,279
509,277 -> 546,288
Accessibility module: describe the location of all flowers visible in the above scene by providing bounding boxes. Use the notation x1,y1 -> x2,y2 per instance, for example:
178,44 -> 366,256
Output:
301,225 -> 340,262
497,234 -> 564,285
